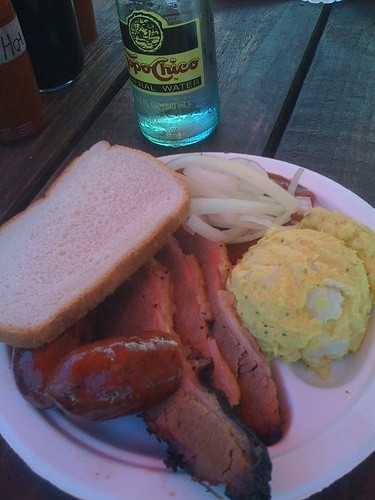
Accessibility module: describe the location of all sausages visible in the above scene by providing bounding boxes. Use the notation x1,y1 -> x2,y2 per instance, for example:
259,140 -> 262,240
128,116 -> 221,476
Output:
46,332 -> 187,421
12,313 -> 98,410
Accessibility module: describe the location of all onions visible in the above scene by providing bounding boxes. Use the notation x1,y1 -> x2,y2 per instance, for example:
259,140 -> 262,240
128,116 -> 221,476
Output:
164,153 -> 312,243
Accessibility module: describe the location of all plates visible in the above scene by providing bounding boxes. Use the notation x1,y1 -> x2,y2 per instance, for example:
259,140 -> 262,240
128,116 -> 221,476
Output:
0,151 -> 373,500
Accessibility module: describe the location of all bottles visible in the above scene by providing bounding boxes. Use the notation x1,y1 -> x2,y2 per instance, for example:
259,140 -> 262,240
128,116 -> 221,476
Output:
74,1 -> 98,44
115,0 -> 220,146
0,0 -> 48,143
13,0 -> 86,91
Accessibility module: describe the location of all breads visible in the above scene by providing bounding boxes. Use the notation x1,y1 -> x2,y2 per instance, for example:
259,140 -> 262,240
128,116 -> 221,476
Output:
0,139 -> 190,348
